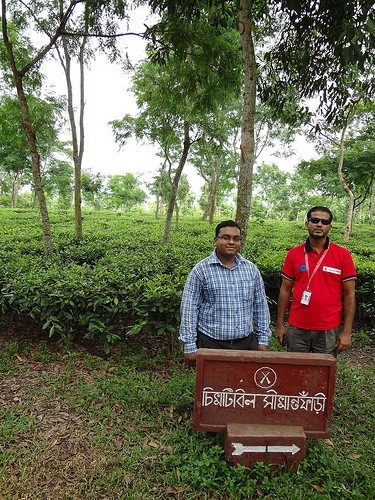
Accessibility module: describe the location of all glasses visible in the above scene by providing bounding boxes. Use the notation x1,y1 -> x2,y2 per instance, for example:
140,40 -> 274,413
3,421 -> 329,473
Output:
218,234 -> 242,242
308,217 -> 331,225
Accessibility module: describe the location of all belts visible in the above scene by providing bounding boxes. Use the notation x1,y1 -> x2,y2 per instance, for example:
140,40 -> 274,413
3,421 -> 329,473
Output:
196,329 -> 252,345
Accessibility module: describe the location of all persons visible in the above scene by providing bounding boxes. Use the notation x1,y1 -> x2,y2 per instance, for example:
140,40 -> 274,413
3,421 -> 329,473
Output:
275,206 -> 355,357
179,220 -> 271,366
